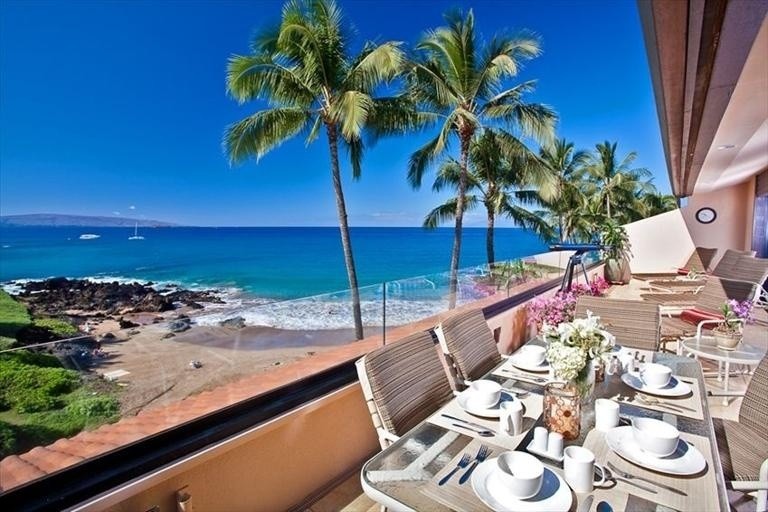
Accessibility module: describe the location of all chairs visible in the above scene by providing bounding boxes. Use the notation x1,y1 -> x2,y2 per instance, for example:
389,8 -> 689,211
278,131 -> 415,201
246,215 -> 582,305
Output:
352,247 -> 768,512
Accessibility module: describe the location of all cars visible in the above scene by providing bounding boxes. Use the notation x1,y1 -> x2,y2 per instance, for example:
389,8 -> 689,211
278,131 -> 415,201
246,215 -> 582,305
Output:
562,445 -> 605,494
541,380 -> 581,441
621,356 -> 635,373
594,399 -> 619,434
498,399 -> 522,436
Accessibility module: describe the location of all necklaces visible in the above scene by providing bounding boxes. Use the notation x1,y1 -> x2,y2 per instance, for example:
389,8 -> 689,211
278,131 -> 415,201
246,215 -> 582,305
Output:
126,220 -> 145,241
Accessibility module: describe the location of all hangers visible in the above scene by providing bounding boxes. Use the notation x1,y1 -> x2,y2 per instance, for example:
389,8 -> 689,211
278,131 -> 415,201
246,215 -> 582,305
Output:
638,393 -> 696,413
451,423 -> 491,436
511,374 -> 545,383
602,466 -> 658,494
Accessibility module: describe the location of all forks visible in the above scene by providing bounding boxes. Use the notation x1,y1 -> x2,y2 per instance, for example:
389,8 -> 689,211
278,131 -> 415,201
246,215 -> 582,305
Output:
605,460 -> 687,497
634,398 -> 683,414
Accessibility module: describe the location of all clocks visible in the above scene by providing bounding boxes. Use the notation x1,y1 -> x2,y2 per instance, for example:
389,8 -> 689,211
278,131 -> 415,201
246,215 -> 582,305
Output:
695,207 -> 717,224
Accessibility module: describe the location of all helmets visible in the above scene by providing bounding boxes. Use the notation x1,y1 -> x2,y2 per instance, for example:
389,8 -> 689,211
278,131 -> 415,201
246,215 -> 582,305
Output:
507,355 -> 549,372
526,439 -> 563,463
605,425 -> 707,478
456,389 -> 523,418
619,371 -> 691,397
471,458 -> 573,512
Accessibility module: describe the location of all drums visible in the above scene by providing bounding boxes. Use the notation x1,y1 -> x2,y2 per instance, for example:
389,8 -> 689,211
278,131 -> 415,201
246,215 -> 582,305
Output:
440,414 -> 495,433
438,453 -> 469,486
502,369 -> 547,380
577,495 -> 593,512
457,445 -> 488,486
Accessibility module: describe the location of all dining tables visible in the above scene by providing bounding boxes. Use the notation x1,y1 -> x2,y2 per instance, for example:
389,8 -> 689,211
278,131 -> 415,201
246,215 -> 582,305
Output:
599,223 -> 632,285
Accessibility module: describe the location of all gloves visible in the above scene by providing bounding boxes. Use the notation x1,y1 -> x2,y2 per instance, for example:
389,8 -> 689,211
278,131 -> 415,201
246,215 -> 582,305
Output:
77,233 -> 99,241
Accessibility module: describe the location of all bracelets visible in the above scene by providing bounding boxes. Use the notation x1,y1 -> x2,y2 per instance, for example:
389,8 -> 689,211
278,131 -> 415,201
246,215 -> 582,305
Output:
495,450 -> 544,501
520,344 -> 546,366
630,416 -> 680,457
638,364 -> 672,389
468,379 -> 501,409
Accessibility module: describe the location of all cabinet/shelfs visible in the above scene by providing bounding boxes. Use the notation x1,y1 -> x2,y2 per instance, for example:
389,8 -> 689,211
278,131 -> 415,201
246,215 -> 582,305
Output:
533,426 -> 548,452
547,432 -> 563,458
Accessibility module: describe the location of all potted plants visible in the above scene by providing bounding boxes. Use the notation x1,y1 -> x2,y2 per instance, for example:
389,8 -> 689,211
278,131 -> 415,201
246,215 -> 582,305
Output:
599,223 -> 632,285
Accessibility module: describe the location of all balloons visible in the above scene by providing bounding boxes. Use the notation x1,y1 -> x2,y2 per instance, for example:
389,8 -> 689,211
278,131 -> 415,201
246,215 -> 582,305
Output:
695,207 -> 717,224
